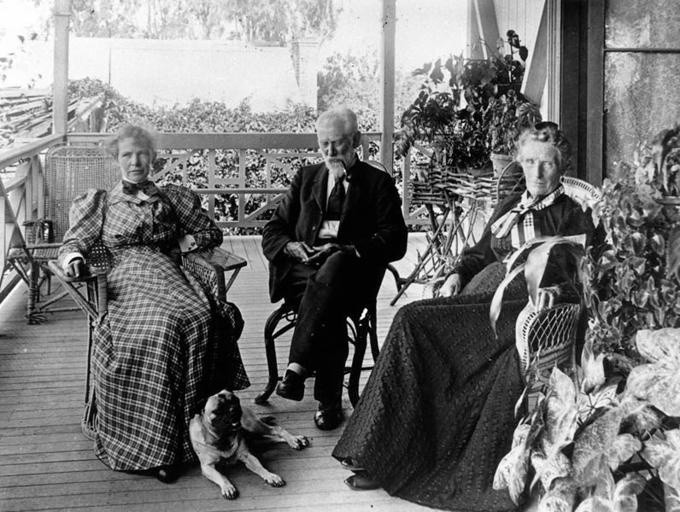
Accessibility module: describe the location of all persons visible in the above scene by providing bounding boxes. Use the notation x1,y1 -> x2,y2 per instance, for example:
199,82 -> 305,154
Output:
57,125 -> 251,484
261,107 -> 407,431
331,121 -> 597,512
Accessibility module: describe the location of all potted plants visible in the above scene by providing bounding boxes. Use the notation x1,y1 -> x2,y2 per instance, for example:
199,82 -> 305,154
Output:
484,91 -> 542,176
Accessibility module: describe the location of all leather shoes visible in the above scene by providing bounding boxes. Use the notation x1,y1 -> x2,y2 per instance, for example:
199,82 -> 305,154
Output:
276,369 -> 306,401
340,458 -> 364,470
344,474 -> 383,490
157,465 -> 180,485
315,399 -> 346,430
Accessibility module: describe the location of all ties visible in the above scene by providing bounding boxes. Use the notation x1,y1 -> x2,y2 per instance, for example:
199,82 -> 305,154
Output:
327,175 -> 345,220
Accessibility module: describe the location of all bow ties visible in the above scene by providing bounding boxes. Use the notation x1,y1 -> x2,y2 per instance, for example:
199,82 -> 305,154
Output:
122,180 -> 160,197
490,182 -> 565,249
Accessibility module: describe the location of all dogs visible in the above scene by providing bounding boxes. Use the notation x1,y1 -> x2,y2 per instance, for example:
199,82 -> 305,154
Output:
188,390 -> 309,500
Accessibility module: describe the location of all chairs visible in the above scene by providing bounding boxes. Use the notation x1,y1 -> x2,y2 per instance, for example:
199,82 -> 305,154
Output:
49,236 -> 247,443
8,144 -> 122,323
255,160 -> 387,413
424,176 -> 600,413
